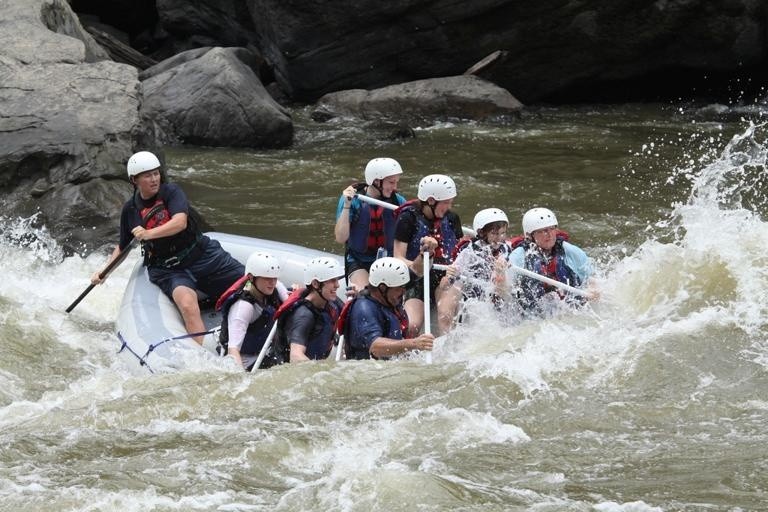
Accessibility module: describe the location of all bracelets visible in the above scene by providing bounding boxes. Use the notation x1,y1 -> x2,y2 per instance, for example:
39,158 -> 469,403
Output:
343,206 -> 351,210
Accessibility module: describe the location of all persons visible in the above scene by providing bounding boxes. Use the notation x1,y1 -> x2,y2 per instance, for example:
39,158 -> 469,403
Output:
438,207 -> 517,314
270,256 -> 358,364
214,250 -> 306,374
392,174 -> 465,338
334,158 -> 408,295
90,150 -> 245,353
492,207 -> 602,324
344,255 -> 436,365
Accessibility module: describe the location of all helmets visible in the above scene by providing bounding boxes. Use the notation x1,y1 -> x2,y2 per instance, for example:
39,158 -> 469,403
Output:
127,151 -> 161,177
522,207 -> 557,238
473,207 -> 509,235
417,174 -> 457,202
245,252 -> 410,287
365,158 -> 403,186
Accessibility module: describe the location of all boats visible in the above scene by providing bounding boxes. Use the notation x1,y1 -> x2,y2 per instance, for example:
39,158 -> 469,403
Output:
116,232 -> 508,384
